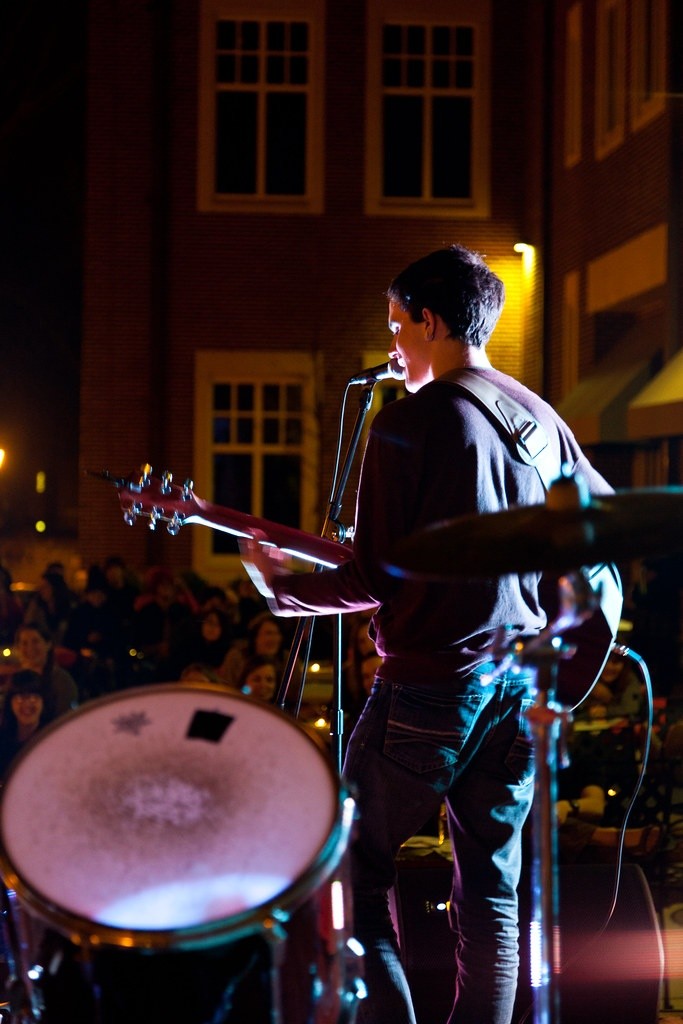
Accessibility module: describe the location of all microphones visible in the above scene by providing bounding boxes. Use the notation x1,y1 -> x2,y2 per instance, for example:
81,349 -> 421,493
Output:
347,359 -> 406,385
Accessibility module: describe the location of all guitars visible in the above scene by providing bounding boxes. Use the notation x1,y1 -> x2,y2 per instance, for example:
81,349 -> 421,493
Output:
117,462 -> 628,716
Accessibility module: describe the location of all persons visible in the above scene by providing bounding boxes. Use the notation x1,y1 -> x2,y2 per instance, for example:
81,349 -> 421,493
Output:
0,554 -> 682,860
240,241 -> 623,1024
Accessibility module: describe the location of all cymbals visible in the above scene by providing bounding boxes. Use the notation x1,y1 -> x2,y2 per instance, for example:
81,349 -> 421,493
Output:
374,485 -> 683,580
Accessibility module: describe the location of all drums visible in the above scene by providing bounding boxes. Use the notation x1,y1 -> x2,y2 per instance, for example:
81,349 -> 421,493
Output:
1,680 -> 359,1024
